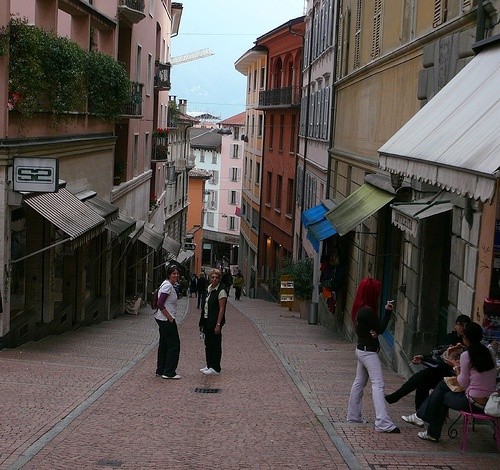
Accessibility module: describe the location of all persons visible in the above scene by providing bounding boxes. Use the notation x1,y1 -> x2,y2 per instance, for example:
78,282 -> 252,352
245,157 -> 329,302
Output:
401,319 -> 496,441
347,274 -> 401,433
175,269 -> 232,298
151,267 -> 182,379
385,314 -> 470,413
197,269 -> 227,375
233,269 -> 244,300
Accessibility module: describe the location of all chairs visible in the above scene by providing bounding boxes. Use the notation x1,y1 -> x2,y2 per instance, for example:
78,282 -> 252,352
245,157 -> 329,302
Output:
447,385 -> 500,454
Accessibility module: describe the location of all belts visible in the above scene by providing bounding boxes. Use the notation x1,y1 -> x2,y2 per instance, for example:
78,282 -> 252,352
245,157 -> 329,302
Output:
466,393 -> 484,410
358,345 -> 377,353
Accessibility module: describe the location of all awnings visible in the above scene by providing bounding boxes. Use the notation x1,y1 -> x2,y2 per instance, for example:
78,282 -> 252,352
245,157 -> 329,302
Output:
376,46 -> 500,206
324,171 -> 409,237
139,223 -> 195,266
75,190 -> 120,227
390,190 -> 453,239
127,217 -> 145,245
303,198 -> 339,253
106,212 -> 137,243
24,186 -> 105,251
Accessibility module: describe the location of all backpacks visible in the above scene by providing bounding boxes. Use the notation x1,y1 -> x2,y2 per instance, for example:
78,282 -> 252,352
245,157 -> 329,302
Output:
151,282 -> 168,310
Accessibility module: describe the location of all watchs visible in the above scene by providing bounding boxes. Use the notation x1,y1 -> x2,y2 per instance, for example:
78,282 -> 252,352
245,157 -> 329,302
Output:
452,366 -> 459,370
217,322 -> 221,326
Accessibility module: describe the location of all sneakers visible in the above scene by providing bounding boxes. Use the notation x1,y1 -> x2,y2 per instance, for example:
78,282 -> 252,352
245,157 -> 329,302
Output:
418,430 -> 438,442
402,413 -> 424,428
203,368 -> 220,376
200,367 -> 209,372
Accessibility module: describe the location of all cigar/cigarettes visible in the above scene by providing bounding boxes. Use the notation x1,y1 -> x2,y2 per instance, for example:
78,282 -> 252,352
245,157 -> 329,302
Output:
410,359 -> 415,365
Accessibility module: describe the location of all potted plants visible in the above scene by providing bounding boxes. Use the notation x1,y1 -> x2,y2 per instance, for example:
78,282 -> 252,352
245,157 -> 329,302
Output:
276,258 -> 314,319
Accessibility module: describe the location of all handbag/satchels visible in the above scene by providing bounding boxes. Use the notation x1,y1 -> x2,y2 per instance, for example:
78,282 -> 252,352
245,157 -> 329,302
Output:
484,392 -> 500,417
233,282 -> 236,288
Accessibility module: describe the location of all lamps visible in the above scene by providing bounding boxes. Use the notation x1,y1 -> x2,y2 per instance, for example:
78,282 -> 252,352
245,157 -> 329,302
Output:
390,173 -> 404,193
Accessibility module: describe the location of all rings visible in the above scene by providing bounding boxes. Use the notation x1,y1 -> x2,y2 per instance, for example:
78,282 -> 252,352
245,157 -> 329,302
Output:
372,334 -> 374,336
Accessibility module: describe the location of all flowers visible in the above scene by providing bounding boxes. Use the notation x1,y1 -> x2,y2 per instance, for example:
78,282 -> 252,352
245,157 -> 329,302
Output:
156,143 -> 170,156
152,128 -> 171,138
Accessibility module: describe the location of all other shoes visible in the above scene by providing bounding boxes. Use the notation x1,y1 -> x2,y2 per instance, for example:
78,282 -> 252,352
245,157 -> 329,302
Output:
384,393 -> 399,404
162,374 -> 181,379
392,428 -> 401,433
